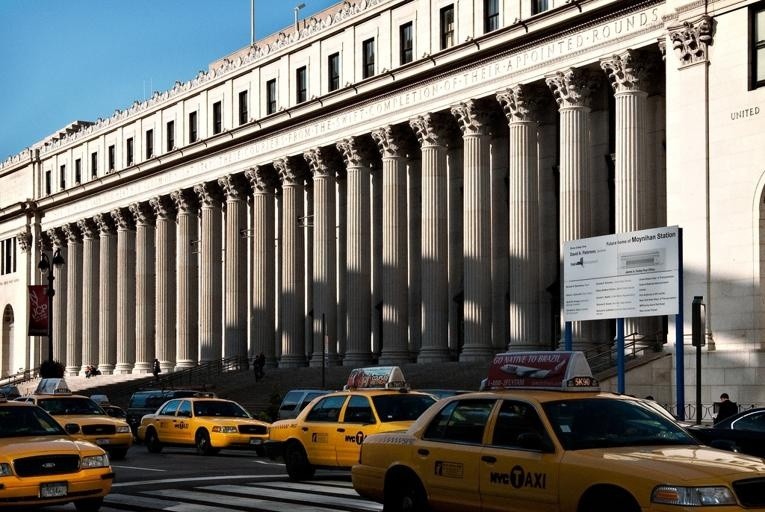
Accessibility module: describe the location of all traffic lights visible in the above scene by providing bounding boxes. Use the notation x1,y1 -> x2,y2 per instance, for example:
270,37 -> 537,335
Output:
692,296 -> 706,346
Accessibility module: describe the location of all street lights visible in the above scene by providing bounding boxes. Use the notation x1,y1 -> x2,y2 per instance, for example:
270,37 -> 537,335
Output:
38,248 -> 65,362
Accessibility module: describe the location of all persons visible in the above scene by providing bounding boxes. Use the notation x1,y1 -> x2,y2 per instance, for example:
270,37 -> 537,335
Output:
86,366 -> 97,378
253,355 -> 263,382
259,352 -> 265,378
712,395 -> 737,425
269,395 -> 282,416
152,358 -> 161,378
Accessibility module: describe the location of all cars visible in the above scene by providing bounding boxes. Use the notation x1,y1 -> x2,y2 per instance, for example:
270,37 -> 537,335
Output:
0,377 -> 271,511
